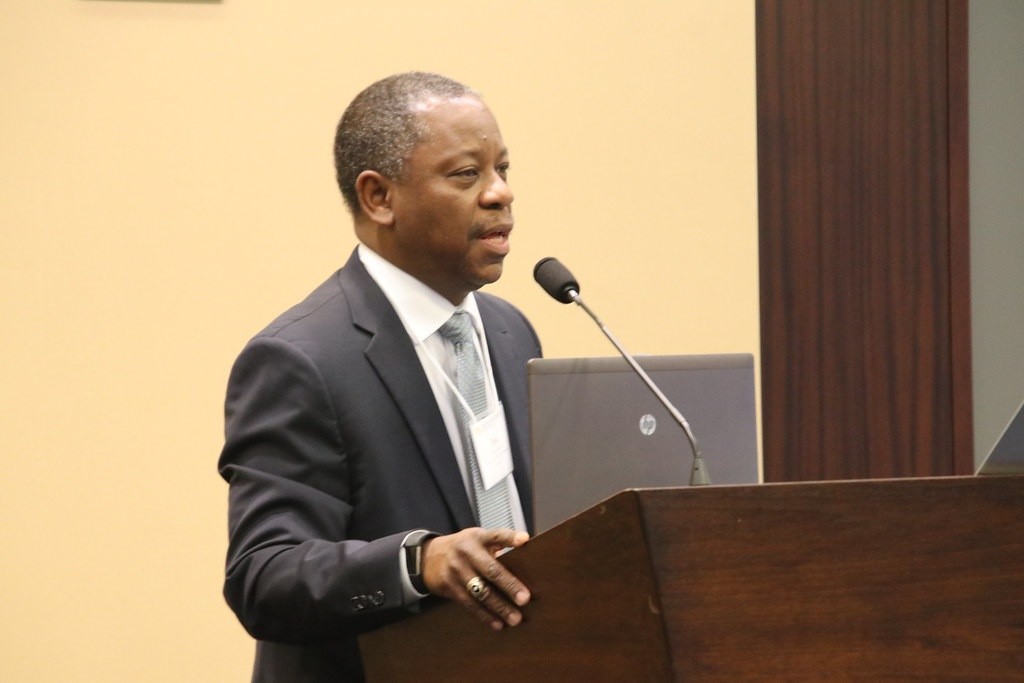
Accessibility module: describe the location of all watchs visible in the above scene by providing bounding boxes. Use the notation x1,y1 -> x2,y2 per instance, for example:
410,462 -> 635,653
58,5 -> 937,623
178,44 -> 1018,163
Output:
403,531 -> 446,595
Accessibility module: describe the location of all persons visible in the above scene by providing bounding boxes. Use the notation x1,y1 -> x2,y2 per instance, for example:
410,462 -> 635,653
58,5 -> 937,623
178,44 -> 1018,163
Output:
216,72 -> 541,683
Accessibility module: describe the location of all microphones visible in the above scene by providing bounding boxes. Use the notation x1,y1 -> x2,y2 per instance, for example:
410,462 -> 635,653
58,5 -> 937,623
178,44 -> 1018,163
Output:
533,257 -> 713,487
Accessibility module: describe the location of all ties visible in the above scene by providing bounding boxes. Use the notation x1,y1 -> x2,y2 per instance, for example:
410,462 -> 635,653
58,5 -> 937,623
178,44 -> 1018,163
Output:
436,311 -> 516,559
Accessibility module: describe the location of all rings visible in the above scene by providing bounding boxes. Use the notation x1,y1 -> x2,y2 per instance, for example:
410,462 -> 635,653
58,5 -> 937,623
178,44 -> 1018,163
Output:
466,574 -> 491,602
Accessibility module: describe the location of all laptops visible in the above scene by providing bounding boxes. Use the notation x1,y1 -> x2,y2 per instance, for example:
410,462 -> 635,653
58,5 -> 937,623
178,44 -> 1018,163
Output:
527,352 -> 759,538
